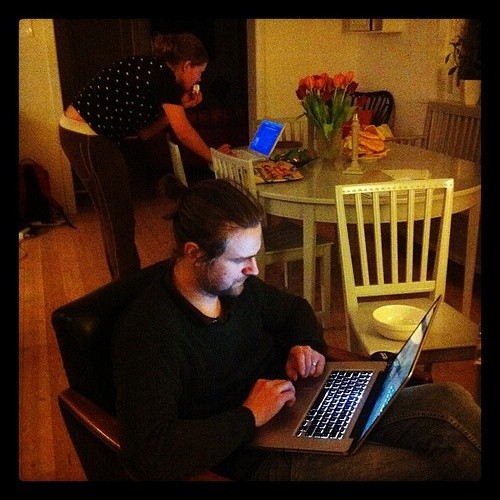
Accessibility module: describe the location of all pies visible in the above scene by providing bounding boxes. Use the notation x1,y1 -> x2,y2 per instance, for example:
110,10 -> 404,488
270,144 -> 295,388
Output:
344,125 -> 384,155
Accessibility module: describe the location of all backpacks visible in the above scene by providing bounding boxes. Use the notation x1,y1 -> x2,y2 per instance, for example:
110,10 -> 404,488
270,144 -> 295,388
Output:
19,157 -> 78,233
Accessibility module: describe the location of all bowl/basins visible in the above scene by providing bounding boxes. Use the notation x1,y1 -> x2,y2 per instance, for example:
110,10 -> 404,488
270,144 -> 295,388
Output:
270,156 -> 320,168
373,305 -> 426,341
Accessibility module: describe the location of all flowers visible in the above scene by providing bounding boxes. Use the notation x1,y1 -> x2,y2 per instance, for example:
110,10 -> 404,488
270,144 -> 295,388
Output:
294,68 -> 366,150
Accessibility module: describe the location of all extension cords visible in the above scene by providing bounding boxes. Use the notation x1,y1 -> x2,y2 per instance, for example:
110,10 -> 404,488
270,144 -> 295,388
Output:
18,227 -> 31,240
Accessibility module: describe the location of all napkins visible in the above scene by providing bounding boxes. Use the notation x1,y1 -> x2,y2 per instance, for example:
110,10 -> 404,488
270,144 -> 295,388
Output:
385,168 -> 430,180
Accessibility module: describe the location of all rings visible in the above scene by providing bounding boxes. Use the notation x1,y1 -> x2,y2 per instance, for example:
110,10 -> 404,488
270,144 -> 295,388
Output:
312,361 -> 317,366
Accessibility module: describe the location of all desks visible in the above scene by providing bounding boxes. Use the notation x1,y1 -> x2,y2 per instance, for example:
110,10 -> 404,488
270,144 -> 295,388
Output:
228,142 -> 480,330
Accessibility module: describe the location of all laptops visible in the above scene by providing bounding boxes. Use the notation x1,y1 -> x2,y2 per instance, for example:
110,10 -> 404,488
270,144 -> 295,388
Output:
232,115 -> 286,163
244,295 -> 443,456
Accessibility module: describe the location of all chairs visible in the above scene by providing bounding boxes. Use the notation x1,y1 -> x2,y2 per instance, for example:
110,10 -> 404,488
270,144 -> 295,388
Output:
162,89 -> 483,385
50,257 -> 430,481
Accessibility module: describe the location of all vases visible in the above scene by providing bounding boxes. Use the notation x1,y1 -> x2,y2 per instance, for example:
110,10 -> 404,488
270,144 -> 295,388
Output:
315,128 -> 342,162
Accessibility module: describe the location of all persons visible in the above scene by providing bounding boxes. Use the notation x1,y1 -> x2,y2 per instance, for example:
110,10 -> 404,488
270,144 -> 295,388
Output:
59,38 -> 236,283
103,178 -> 331,500
